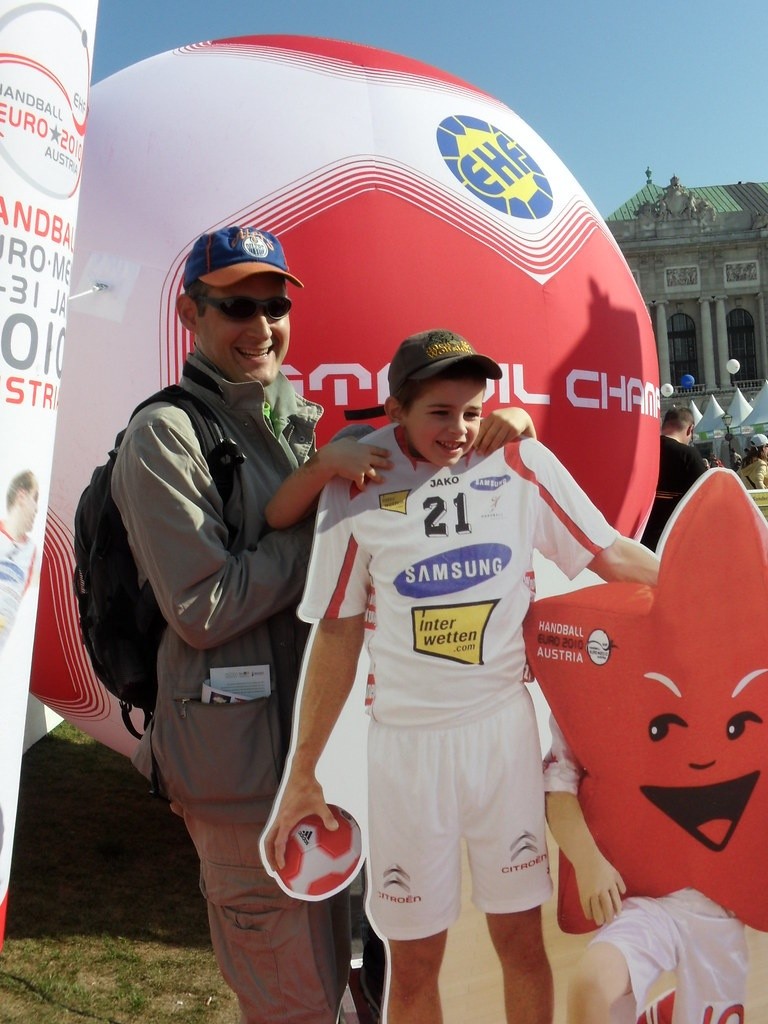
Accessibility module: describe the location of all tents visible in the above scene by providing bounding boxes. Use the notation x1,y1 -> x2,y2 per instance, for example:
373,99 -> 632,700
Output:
673,380 -> 768,434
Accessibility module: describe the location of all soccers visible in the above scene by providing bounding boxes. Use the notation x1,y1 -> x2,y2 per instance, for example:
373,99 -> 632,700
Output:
270,802 -> 363,898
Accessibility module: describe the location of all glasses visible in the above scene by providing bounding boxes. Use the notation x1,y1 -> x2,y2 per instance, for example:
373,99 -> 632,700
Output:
193,296 -> 291,319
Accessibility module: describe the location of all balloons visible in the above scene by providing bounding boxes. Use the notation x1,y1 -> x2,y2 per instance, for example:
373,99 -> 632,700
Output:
29,34 -> 658,778
522,467 -> 768,935
28,33 -> 661,759
0,1 -> 98,903
661,383 -> 674,399
680,374 -> 696,389
726,358 -> 741,375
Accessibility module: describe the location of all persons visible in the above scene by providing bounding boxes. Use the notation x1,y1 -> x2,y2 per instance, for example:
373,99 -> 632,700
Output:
0,470 -> 39,656
110,226 -> 660,1024
542,708 -> 768,1024
640,408 -> 768,553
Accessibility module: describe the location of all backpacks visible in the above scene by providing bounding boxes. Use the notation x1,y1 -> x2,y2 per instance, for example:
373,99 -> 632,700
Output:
71,384 -> 249,740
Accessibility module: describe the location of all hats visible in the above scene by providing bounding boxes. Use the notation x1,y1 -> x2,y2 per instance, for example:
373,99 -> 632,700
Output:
750,434 -> 768,451
388,329 -> 502,396
181,227 -> 303,288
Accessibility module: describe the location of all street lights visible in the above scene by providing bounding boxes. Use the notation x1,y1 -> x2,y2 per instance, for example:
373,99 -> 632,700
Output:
721,409 -> 735,470
